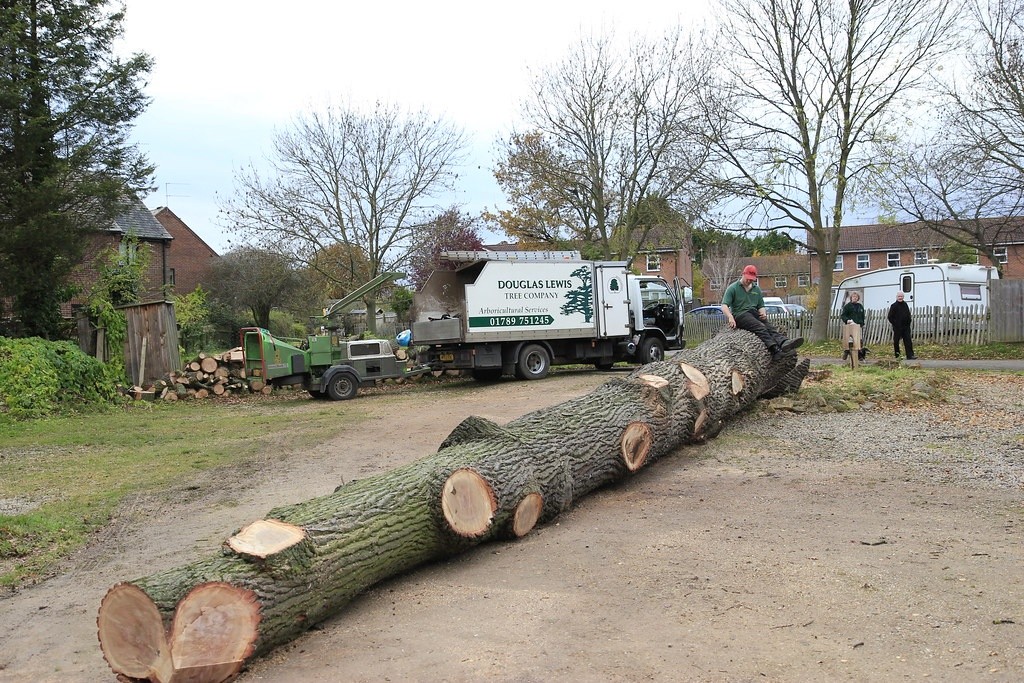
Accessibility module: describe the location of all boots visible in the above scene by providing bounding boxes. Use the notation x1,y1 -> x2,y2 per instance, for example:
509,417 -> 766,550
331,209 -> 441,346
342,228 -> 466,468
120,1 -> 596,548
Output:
771,336 -> 805,362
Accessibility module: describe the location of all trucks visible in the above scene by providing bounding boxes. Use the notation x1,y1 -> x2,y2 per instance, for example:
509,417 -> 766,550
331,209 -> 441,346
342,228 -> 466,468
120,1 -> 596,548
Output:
410,250 -> 687,381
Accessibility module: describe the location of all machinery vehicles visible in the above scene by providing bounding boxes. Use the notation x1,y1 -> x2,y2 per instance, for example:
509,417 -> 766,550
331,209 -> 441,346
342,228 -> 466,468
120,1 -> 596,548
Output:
240,271 -> 432,403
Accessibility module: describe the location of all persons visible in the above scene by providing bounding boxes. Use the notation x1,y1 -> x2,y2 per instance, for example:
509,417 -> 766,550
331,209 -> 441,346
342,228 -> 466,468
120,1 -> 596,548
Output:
841,292 -> 865,360
887,291 -> 919,360
721,265 -> 804,360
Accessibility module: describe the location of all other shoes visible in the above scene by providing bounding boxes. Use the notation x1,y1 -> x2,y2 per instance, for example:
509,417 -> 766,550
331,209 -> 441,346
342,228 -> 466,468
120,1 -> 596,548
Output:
842,350 -> 864,361
895,355 -> 918,360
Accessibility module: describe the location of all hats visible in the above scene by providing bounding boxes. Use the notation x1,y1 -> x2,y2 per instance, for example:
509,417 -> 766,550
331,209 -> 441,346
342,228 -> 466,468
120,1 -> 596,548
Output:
742,264 -> 758,281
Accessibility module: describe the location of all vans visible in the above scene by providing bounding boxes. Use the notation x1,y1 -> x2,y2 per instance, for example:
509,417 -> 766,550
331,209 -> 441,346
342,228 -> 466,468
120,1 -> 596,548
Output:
830,256 -> 1001,340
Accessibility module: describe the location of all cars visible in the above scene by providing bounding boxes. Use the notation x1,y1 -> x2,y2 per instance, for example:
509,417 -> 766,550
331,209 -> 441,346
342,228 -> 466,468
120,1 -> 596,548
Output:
762,296 -> 815,330
683,306 -> 731,328
